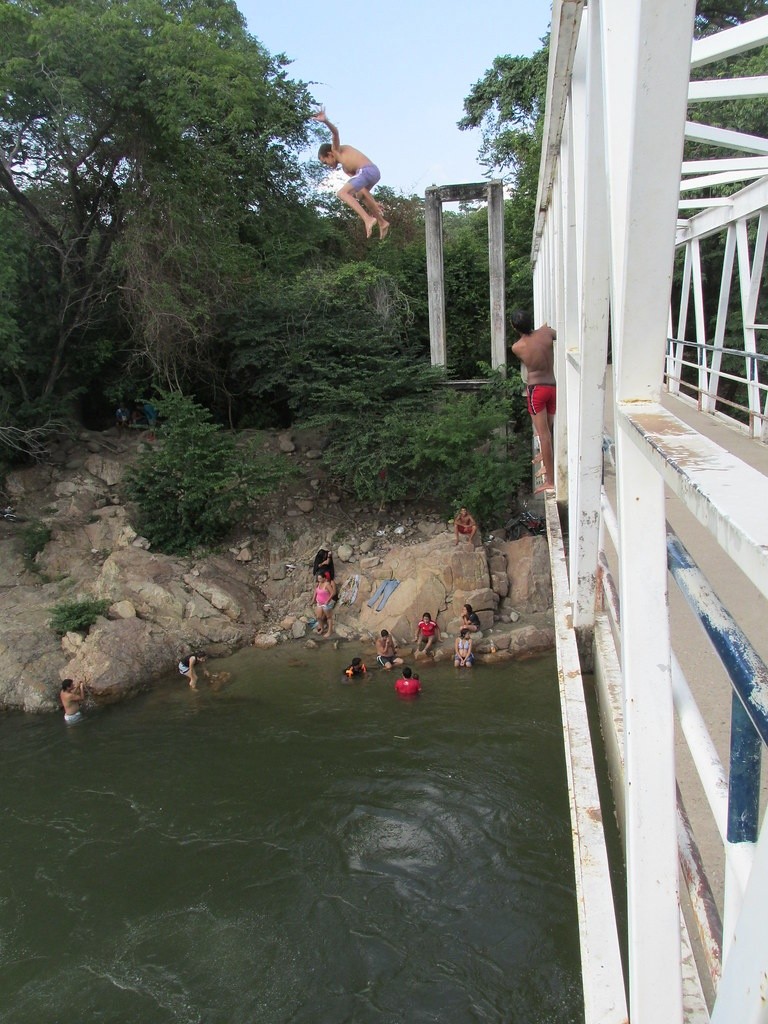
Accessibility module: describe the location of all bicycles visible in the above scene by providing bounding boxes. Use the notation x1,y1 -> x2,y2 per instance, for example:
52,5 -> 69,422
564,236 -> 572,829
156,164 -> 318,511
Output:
504,498 -> 546,541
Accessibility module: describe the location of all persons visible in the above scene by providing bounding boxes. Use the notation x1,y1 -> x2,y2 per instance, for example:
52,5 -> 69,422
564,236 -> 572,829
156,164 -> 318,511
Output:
394,667 -> 420,693
313,103 -> 390,239
412,612 -> 440,658
179,650 -> 206,688
453,507 -> 476,545
460,604 -> 481,632
376,630 -> 403,668
344,657 -> 366,679
313,541 -> 335,579
60,679 -> 85,721
454,628 -> 474,667
511,310 -> 557,493
310,572 -> 338,637
